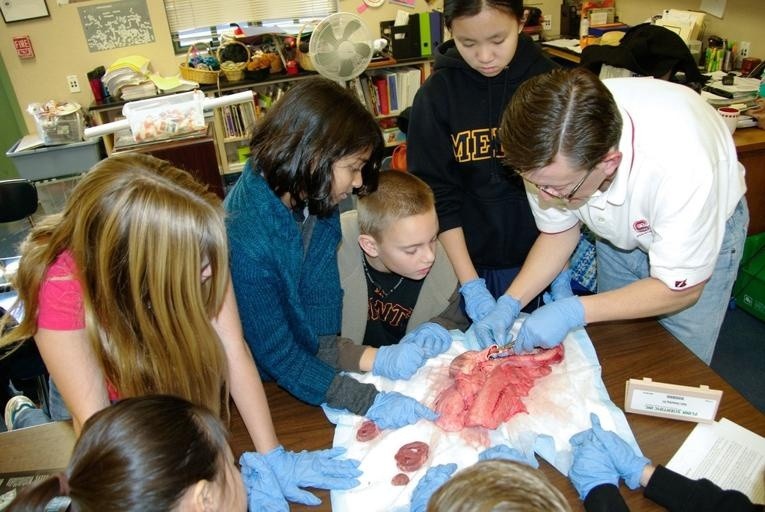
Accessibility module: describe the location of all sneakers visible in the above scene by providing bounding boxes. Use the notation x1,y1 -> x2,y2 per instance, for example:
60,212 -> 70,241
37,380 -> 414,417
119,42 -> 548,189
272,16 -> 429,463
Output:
3,395 -> 38,432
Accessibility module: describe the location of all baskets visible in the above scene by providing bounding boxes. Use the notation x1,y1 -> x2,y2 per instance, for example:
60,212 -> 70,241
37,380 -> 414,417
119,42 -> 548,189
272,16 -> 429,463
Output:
294,23 -> 317,72
178,41 -> 221,84
215,41 -> 250,81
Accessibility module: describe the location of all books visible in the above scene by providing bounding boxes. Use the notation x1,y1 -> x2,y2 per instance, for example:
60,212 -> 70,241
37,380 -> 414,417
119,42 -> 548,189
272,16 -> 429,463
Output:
98,57 -> 157,102
214,62 -> 439,141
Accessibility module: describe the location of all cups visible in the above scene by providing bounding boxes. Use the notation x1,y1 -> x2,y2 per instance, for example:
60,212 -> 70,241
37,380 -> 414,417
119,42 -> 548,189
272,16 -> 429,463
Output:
718,107 -> 740,136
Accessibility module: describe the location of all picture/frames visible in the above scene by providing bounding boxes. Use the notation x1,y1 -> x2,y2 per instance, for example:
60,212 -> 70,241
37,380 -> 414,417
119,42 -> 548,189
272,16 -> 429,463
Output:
1,1 -> 51,27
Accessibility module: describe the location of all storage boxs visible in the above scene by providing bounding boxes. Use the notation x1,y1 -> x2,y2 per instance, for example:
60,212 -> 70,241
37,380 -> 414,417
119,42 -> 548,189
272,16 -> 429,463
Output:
8,89 -> 203,212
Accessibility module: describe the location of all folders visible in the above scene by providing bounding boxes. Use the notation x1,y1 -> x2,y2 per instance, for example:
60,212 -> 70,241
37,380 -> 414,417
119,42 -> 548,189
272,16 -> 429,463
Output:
403,7 -> 441,55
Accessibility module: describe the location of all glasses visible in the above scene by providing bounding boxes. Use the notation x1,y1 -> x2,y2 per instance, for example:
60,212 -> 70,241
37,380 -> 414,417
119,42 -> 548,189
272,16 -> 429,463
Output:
512,168 -> 593,202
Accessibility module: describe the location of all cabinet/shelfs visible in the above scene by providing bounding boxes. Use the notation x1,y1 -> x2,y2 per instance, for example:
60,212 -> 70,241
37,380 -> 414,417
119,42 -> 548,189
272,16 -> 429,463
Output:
94,61 -> 435,176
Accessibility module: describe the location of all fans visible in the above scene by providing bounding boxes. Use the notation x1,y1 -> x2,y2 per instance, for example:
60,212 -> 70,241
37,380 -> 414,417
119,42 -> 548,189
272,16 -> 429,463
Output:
309,13 -> 374,81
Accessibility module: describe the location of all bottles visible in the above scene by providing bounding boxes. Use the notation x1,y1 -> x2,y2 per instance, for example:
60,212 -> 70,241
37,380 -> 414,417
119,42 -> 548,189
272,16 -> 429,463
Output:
88,65 -> 109,103
579,17 -> 589,40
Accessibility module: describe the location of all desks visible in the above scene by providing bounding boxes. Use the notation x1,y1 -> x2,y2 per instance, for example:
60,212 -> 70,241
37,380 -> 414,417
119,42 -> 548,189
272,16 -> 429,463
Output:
545,40 -> 764,232
216,318 -> 765,509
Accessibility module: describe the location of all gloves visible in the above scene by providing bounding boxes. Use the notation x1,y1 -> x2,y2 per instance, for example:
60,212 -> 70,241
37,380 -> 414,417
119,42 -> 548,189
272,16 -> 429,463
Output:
568,412 -> 651,491
238,451 -> 290,512
478,444 -> 540,472
568,432 -> 620,501
410,462 -> 457,512
372,268 -> 586,381
364,390 -> 438,430
262,443 -> 361,505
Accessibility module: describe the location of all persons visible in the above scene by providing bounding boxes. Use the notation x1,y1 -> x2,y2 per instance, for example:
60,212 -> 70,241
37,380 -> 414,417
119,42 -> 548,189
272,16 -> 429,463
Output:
219,74 -> 443,433
472,67 -> 751,367
410,443 -> 574,511
0,150 -> 365,507
568,412 -> 765,512
315,170 -> 470,382
408,0 -> 577,329
7,393 -> 293,512
